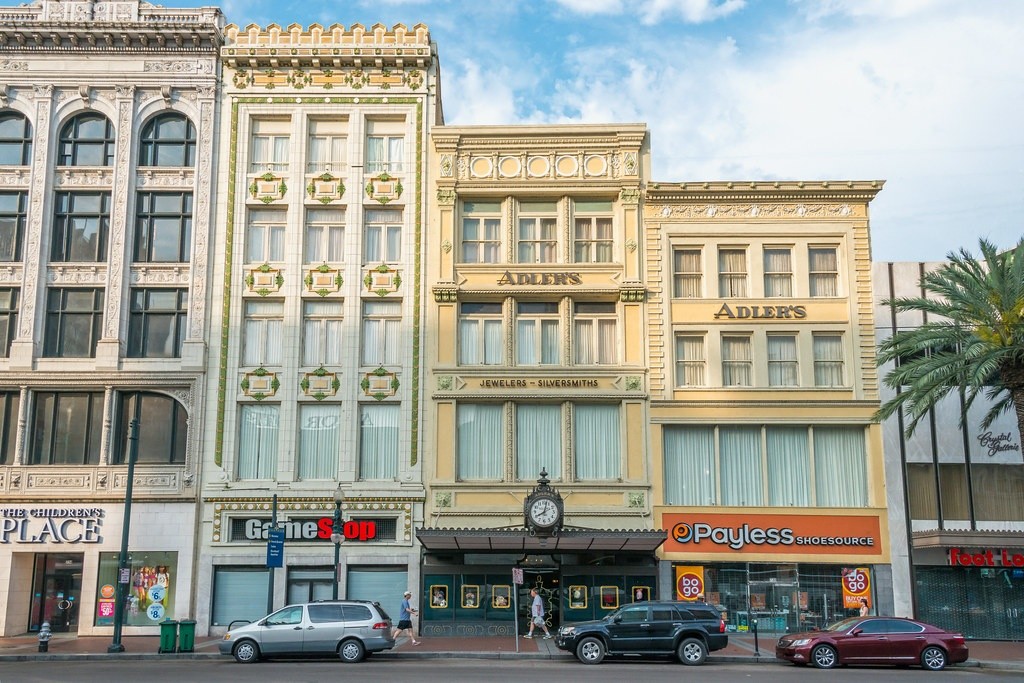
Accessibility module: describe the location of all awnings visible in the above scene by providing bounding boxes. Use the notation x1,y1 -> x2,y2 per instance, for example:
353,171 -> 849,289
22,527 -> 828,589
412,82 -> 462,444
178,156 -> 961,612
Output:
415,527 -> 669,557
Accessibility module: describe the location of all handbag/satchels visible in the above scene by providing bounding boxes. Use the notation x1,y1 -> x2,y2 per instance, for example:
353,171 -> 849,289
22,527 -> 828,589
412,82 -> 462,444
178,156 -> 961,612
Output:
534,616 -> 545,628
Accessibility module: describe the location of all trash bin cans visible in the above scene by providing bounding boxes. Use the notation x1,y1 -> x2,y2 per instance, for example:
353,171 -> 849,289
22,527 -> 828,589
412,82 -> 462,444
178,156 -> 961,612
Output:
178,619 -> 197,653
157,619 -> 179,653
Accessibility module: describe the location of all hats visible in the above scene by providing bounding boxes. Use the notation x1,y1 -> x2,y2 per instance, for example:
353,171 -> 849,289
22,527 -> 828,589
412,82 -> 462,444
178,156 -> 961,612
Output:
404,591 -> 413,596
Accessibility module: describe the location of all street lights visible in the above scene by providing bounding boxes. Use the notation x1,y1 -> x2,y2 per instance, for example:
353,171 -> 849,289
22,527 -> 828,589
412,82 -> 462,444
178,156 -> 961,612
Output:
331,483 -> 344,599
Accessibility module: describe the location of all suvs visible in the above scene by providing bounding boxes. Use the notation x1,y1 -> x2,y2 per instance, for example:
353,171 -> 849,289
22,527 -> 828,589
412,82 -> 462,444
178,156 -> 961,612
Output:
554,599 -> 729,666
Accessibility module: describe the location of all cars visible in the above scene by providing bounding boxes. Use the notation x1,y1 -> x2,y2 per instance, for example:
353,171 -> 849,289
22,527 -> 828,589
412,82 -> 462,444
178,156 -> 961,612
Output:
774,615 -> 970,672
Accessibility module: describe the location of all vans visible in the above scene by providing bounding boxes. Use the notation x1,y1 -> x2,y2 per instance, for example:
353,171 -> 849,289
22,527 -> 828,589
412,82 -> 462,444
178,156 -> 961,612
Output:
219,599 -> 396,663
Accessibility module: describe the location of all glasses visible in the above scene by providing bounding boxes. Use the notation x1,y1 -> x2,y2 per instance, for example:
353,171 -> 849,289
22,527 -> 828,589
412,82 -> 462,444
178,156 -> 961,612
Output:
861,601 -> 863,603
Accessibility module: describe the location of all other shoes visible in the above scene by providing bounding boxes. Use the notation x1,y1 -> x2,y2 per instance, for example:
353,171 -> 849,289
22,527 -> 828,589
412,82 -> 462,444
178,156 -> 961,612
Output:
543,635 -> 552,640
524,635 -> 532,639
412,641 -> 421,645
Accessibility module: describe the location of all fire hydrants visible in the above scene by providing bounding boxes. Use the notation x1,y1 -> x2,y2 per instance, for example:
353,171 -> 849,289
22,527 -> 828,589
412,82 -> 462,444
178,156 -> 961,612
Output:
36,621 -> 52,652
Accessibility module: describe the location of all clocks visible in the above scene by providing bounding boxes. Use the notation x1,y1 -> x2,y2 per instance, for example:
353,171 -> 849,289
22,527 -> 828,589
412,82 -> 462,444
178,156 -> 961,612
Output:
523,467 -> 564,538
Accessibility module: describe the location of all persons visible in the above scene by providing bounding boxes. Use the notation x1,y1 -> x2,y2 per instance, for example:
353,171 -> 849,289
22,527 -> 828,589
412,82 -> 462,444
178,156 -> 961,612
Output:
859,599 -> 869,617
635,588 -> 643,600
523,588 -> 552,640
392,591 -> 421,646
133,566 -> 169,611
